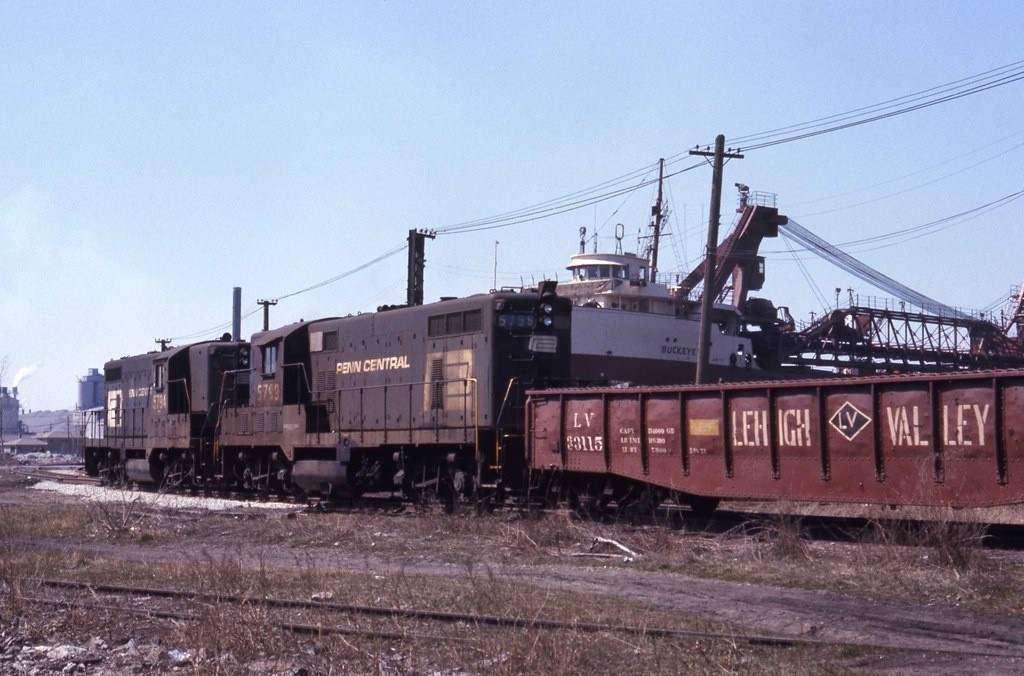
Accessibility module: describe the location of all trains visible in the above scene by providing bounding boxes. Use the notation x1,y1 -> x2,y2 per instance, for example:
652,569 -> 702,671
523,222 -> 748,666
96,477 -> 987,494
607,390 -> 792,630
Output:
82,280 -> 1024,528
